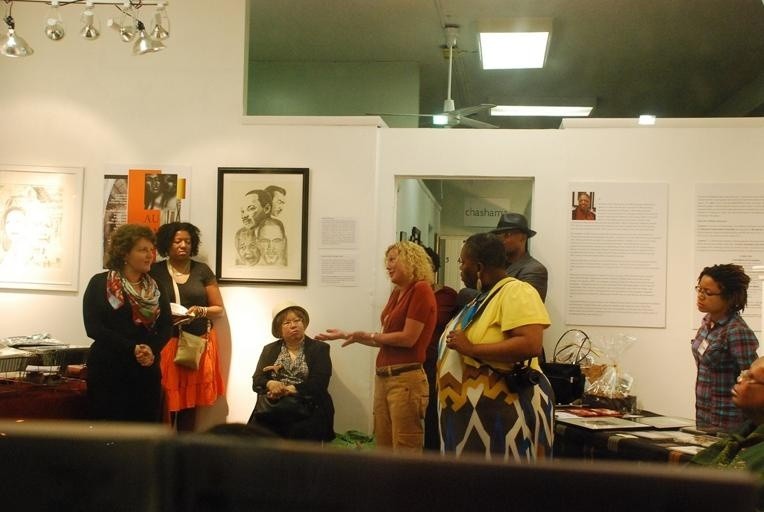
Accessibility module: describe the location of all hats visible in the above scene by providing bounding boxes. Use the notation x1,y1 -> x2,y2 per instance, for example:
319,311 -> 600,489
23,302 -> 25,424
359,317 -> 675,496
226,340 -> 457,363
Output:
271,302 -> 310,338
487,213 -> 537,237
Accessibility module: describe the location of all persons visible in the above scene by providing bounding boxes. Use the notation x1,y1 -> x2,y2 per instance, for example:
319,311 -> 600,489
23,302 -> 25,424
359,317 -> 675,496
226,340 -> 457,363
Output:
233,228 -> 260,265
145,173 -> 163,212
692,263 -> 760,433
78,225 -> 172,422
266,185 -> 286,216
246,300 -> 335,444
490,213 -> 548,303
314,241 -> 437,454
147,222 -> 224,433
257,220 -> 286,269
420,244 -> 458,452
435,233 -> 556,464
240,190 -> 273,229
684,356 -> 764,477
572,192 -> 596,220
162,173 -> 178,219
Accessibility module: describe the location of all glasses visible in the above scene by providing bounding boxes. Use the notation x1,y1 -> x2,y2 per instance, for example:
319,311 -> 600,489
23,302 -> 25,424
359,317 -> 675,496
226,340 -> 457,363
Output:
695,287 -> 722,297
741,369 -> 763,385
385,258 -> 399,265
280,317 -> 304,328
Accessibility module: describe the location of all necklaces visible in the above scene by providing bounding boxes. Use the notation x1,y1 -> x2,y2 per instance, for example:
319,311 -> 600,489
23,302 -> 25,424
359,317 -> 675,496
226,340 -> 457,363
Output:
170,262 -> 190,277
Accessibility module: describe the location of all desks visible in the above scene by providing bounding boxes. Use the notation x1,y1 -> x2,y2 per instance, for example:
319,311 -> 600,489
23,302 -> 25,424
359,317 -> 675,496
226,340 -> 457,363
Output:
0,376 -> 87,422
550,406 -> 736,464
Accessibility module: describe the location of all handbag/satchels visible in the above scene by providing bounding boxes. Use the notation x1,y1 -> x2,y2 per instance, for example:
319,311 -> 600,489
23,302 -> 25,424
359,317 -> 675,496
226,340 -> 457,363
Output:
541,329 -> 592,404
254,394 -> 312,422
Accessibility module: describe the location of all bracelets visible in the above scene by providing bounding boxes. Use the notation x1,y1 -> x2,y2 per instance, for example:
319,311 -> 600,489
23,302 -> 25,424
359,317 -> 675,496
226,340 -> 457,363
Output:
198,306 -> 207,320
370,332 -> 377,347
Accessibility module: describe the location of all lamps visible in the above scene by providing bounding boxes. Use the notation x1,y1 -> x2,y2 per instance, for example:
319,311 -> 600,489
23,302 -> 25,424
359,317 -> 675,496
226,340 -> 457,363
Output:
488,98 -> 596,118
0,1 -> 34,57
132,20 -> 166,58
475,16 -> 555,69
107,3 -> 136,44
150,3 -> 171,40
43,0 -> 64,40
80,1 -> 101,41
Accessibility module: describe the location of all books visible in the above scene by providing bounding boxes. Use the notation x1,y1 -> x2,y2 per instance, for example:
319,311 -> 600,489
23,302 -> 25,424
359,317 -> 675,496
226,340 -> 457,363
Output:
555,411 -> 653,430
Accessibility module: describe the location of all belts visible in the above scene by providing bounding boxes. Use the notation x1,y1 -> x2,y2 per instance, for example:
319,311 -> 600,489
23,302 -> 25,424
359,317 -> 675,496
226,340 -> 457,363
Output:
376,365 -> 421,378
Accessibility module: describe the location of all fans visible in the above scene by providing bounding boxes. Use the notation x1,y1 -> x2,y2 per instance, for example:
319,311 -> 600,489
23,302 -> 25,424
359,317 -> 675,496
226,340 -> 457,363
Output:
363,26 -> 498,130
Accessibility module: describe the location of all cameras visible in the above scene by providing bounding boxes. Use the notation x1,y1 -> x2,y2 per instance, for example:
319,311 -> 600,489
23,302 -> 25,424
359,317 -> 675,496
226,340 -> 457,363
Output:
505,362 -> 542,394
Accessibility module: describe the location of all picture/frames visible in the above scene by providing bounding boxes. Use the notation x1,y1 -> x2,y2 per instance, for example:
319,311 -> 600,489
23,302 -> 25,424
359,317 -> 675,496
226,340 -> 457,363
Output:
215,167 -> 309,288
0,164 -> 86,294
96,162 -> 191,276
